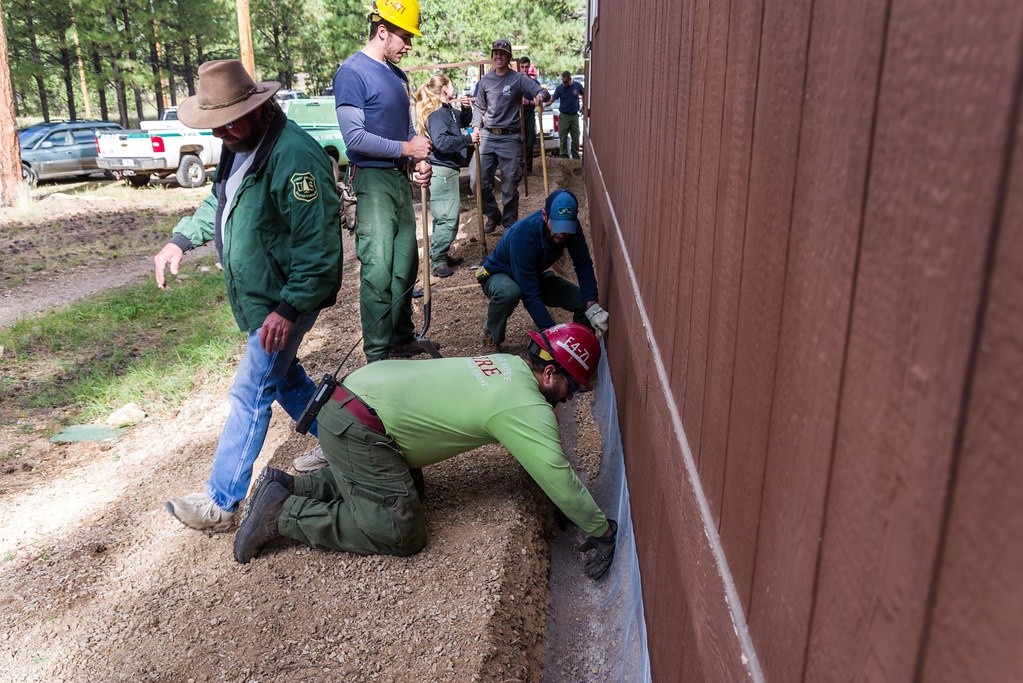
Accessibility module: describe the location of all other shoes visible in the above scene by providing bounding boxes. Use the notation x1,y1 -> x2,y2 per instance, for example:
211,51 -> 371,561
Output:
520,169 -> 529,176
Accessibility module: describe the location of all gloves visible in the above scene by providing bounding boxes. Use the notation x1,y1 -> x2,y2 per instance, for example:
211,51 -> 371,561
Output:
584,303 -> 609,337
578,519 -> 620,579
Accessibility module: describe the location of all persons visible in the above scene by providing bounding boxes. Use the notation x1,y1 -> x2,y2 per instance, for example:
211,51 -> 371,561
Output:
479,188 -> 608,355
414,74 -> 481,278
233,323 -> 620,581
471,40 -> 583,231
154,59 -> 343,533
333,0 -> 440,365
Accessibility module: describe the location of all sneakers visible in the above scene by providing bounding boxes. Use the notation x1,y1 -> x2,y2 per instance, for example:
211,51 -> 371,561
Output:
238,466 -> 294,525
434,264 -> 454,278
389,332 -> 441,358
477,321 -> 500,357
164,492 -> 233,532
233,480 -> 292,563
292,441 -> 329,472
445,254 -> 464,266
503,223 -> 512,235
484,216 -> 502,232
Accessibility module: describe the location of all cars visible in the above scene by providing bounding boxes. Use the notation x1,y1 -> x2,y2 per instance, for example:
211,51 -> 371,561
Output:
17,118 -> 126,186
559,74 -> 585,90
273,88 -> 310,116
532,90 -> 572,157
287,99 -> 351,185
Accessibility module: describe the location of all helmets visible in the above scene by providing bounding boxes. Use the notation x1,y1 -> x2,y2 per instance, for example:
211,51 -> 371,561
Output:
526,321 -> 602,393
363,0 -> 423,38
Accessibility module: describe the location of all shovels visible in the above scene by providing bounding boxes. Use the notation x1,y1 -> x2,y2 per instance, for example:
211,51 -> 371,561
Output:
411,157 -> 443,360
471,141 -> 487,270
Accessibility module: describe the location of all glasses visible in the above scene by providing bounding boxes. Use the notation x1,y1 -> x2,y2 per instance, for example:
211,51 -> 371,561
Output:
491,41 -> 512,50
520,65 -> 529,69
543,360 -> 581,396
562,78 -> 571,84
218,122 -> 233,131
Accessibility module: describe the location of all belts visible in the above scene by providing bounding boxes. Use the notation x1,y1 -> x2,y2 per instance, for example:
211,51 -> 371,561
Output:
486,127 -> 521,136
389,164 -> 408,171
331,387 -> 385,434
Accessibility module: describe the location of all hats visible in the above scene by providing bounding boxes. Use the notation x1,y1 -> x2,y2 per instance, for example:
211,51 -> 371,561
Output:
491,38 -> 514,55
545,188 -> 579,235
177,58 -> 282,129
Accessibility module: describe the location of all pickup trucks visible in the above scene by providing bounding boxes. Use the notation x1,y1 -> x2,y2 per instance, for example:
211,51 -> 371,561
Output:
95,105 -> 224,189
310,86 -> 336,99
